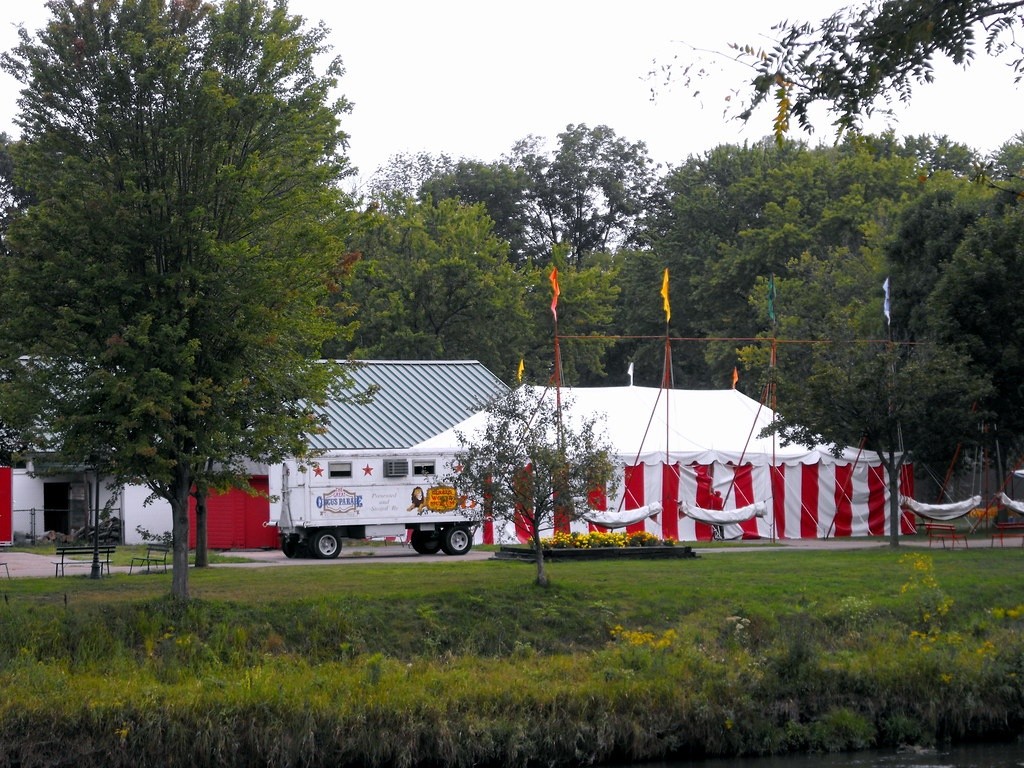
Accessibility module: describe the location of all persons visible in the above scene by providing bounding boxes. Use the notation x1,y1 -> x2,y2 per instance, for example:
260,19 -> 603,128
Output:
711,488 -> 724,540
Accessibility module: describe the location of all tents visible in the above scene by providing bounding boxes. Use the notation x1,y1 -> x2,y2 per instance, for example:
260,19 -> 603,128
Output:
361,385 -> 914,541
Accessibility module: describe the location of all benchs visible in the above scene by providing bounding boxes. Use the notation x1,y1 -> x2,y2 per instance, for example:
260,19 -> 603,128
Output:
51,544 -> 116,578
925,522 -> 968,551
128,544 -> 169,574
991,522 -> 1024,547
